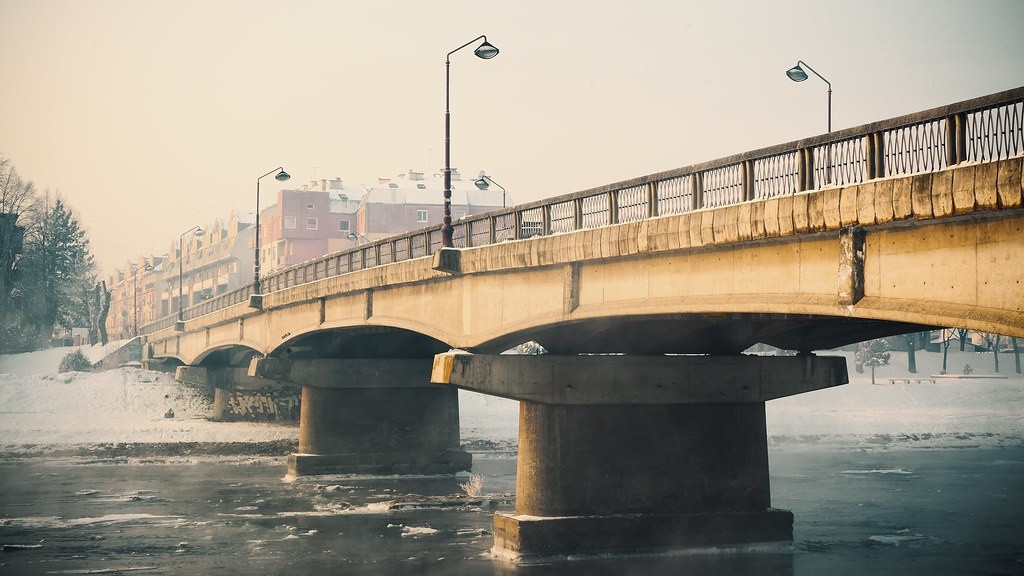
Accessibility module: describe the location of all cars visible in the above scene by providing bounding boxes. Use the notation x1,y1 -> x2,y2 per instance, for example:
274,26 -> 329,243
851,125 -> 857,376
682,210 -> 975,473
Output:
522,220 -> 554,238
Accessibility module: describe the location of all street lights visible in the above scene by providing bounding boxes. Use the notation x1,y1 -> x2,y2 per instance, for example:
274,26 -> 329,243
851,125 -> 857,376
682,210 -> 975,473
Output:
786,60 -> 832,187
440,34 -> 500,248
133,263 -> 154,336
252,166 -> 290,296
474,175 -> 506,229
177,225 -> 206,321
